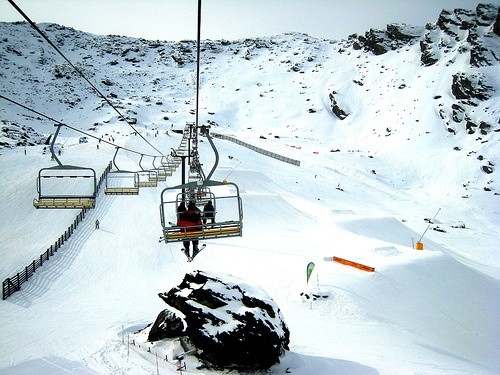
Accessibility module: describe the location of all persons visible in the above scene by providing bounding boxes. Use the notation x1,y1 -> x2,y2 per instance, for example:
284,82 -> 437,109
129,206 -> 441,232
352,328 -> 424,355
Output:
202,200 -> 215,224
176,202 -> 202,256
95,218 -> 100,229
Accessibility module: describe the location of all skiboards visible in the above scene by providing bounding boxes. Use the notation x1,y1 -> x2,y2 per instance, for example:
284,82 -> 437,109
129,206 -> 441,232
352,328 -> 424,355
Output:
181,244 -> 206,262
